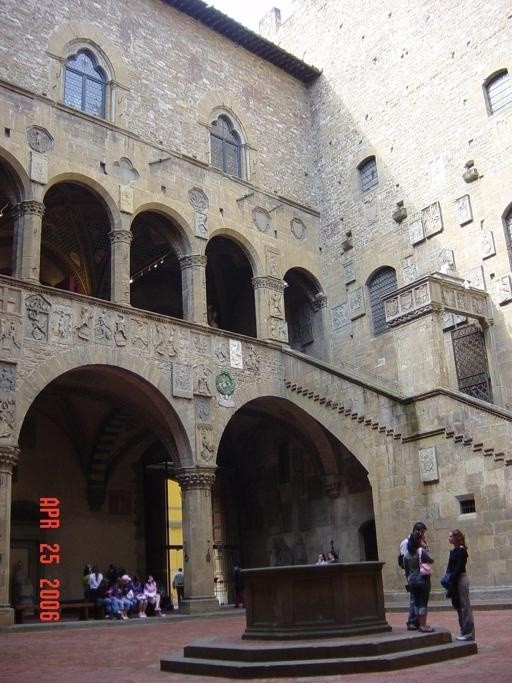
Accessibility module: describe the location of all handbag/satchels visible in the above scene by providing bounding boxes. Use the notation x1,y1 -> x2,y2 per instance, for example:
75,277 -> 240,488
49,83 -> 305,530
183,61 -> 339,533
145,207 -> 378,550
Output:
420,563 -> 432,576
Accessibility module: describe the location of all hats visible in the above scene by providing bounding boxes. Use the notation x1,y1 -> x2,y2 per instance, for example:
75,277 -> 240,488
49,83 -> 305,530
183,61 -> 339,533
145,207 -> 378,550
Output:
122,575 -> 131,581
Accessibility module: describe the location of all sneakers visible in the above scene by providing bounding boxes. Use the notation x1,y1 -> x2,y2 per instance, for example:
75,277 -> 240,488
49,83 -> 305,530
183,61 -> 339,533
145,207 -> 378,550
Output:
154,607 -> 162,611
407,622 -> 435,632
121,613 -> 147,619
457,634 -> 469,641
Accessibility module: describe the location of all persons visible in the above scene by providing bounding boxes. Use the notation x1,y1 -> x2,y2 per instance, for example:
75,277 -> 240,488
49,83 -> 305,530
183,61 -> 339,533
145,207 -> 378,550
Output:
445,526 -> 476,642
80,563 -> 166,620
399,521 -> 429,629
173,567 -> 183,604
315,551 -> 326,564
326,550 -> 340,563
12,559 -> 29,606
230,562 -> 246,608
403,529 -> 436,633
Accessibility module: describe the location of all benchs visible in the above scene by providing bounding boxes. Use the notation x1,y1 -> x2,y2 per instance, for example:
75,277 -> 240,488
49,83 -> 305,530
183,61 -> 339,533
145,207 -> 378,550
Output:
16,601 -> 108,623
